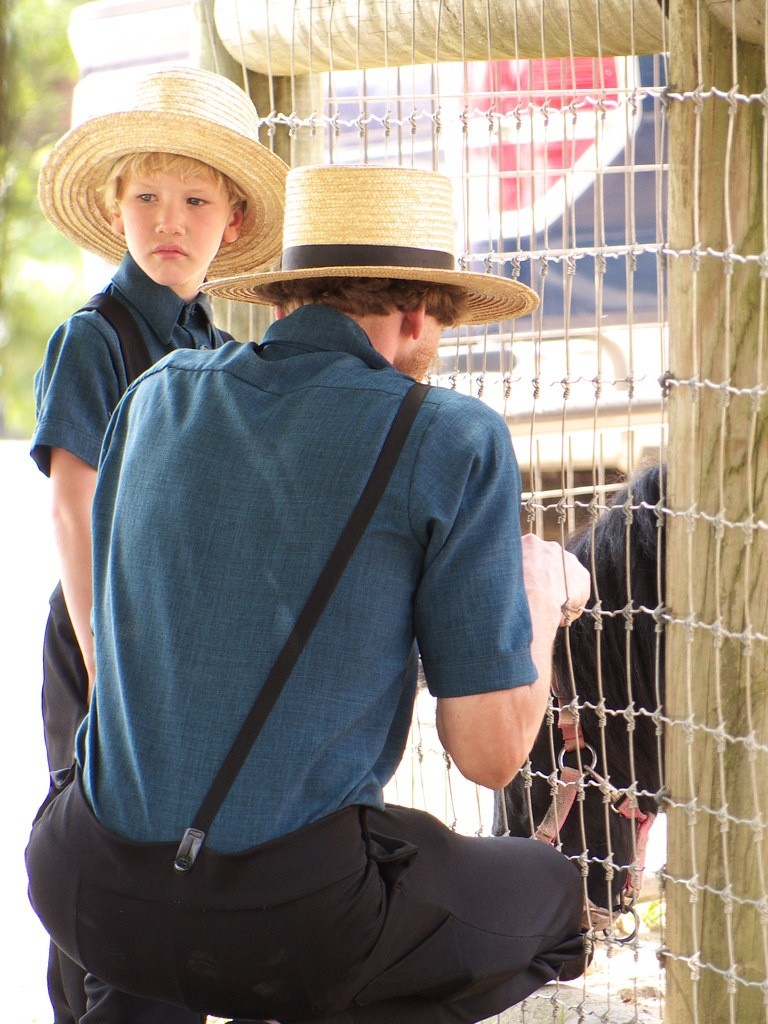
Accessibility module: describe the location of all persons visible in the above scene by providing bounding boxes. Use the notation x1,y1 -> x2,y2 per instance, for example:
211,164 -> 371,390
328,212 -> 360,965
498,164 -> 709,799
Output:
30,68 -> 290,1024
22,164 -> 596,1024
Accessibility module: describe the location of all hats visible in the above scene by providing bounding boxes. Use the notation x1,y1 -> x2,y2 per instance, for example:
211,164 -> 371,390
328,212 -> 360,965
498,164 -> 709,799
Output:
37,66 -> 291,280
197,162 -> 540,324
